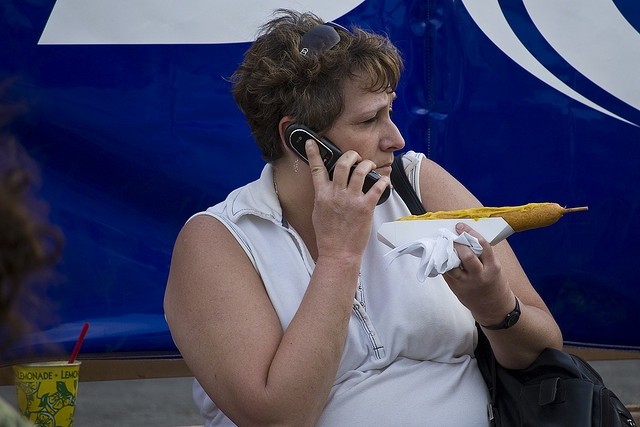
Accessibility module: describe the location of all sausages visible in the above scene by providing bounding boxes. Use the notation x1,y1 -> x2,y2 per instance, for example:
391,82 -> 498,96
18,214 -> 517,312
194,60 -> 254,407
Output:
399,202 -> 566,231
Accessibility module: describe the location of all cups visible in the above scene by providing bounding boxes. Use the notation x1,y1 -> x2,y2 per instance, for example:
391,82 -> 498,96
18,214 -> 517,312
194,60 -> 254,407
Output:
11,359 -> 83,427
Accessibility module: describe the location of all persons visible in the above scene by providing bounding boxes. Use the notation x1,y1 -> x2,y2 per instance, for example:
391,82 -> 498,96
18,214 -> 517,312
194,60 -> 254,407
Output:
163,9 -> 565,427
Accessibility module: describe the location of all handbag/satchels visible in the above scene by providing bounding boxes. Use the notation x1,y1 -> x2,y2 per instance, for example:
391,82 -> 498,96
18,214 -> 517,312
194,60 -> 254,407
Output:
474,320 -> 636,426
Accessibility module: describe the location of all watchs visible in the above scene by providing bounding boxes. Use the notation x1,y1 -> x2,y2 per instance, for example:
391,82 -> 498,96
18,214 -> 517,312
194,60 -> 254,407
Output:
480,296 -> 521,330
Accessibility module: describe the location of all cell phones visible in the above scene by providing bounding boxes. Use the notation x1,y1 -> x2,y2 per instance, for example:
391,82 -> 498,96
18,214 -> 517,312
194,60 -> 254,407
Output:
283,122 -> 390,206
